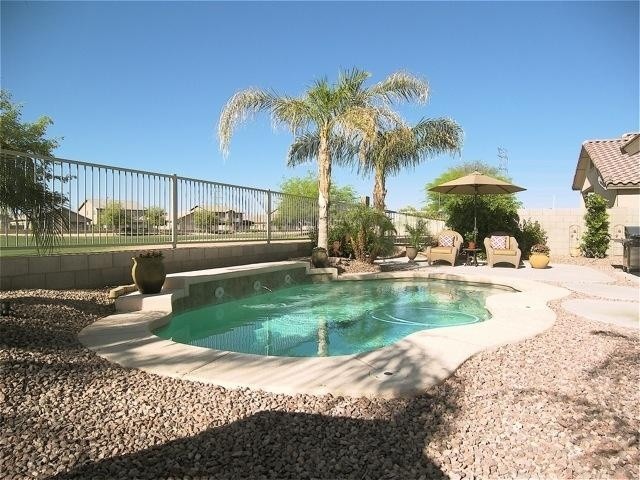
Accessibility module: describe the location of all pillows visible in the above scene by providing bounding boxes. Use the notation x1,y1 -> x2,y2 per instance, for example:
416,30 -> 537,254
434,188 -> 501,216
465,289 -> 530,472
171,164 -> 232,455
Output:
490,236 -> 507,249
439,234 -> 455,247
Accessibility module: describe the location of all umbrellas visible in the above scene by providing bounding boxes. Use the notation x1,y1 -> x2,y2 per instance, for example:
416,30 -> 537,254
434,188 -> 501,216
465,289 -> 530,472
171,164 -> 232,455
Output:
427,171 -> 527,243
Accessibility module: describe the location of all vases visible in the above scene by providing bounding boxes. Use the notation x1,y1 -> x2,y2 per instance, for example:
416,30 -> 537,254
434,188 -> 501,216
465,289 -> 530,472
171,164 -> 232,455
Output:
468,242 -> 476,249
529,252 -> 549,269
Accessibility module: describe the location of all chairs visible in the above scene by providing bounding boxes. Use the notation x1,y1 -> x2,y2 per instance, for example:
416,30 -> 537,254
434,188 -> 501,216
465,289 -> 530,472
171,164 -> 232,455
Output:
484,231 -> 522,270
427,229 -> 464,266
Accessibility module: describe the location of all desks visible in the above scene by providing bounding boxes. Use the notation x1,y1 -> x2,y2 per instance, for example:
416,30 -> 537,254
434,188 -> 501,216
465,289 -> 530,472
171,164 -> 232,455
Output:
463,248 -> 483,267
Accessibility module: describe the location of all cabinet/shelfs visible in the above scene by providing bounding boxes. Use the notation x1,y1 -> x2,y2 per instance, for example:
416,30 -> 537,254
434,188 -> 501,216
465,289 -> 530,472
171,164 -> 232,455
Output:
622,240 -> 640,273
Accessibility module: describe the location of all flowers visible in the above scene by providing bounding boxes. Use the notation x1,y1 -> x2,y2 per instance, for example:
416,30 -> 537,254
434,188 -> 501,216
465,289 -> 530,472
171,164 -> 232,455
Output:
464,231 -> 479,243
528,243 -> 551,253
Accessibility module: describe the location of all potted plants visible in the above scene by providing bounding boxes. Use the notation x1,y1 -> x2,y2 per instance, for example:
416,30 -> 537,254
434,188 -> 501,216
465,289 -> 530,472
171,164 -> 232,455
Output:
312,246 -> 328,268
405,243 -> 418,264
131,252 -> 165,295
